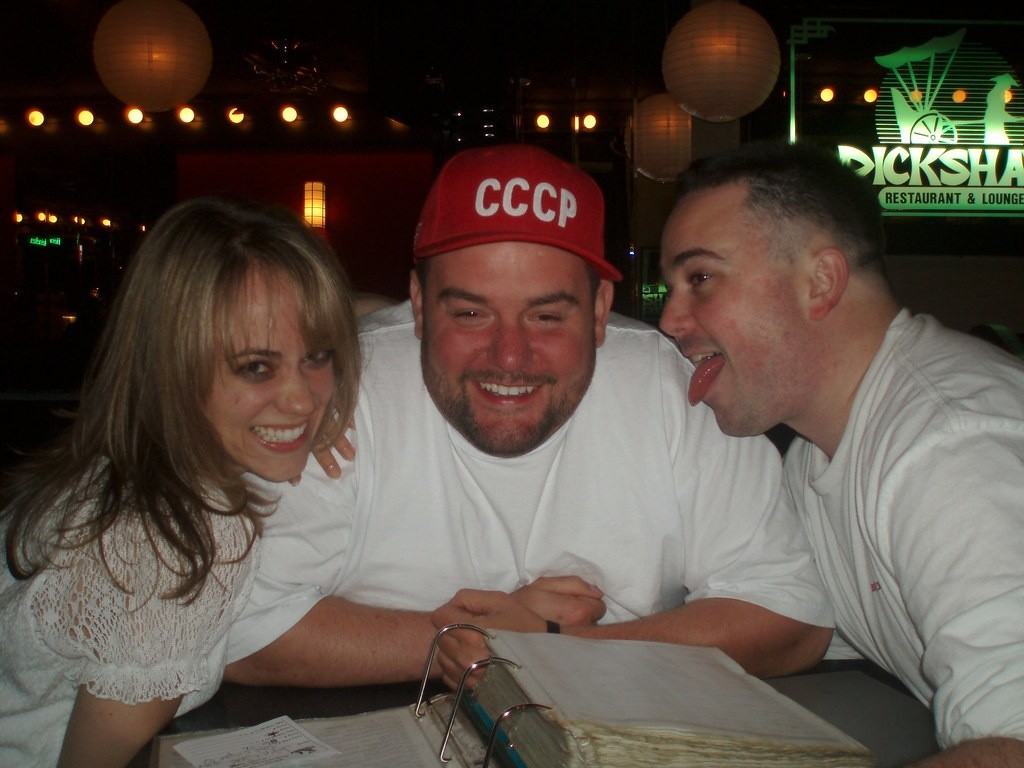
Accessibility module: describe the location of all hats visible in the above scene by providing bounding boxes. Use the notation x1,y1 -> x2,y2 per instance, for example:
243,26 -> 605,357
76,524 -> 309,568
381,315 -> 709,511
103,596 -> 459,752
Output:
412,144 -> 624,283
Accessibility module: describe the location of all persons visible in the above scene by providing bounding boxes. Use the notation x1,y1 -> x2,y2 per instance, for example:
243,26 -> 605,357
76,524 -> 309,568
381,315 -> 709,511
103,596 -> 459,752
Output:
1,204 -> 363,768
431,147 -> 1024,768
222,146 -> 834,685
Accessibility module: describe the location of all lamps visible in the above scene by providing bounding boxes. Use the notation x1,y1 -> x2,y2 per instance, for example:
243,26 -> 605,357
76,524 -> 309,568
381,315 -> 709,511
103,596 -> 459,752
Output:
304,180 -> 328,231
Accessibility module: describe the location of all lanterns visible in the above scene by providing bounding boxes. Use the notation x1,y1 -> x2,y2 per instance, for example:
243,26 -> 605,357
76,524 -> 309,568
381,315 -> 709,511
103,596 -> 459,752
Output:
624,94 -> 693,182
663,1 -> 782,123
94,1 -> 212,113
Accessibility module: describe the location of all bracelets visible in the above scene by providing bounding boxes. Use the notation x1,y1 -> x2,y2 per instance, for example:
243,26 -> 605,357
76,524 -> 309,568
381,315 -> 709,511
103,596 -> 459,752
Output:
548,621 -> 559,634
